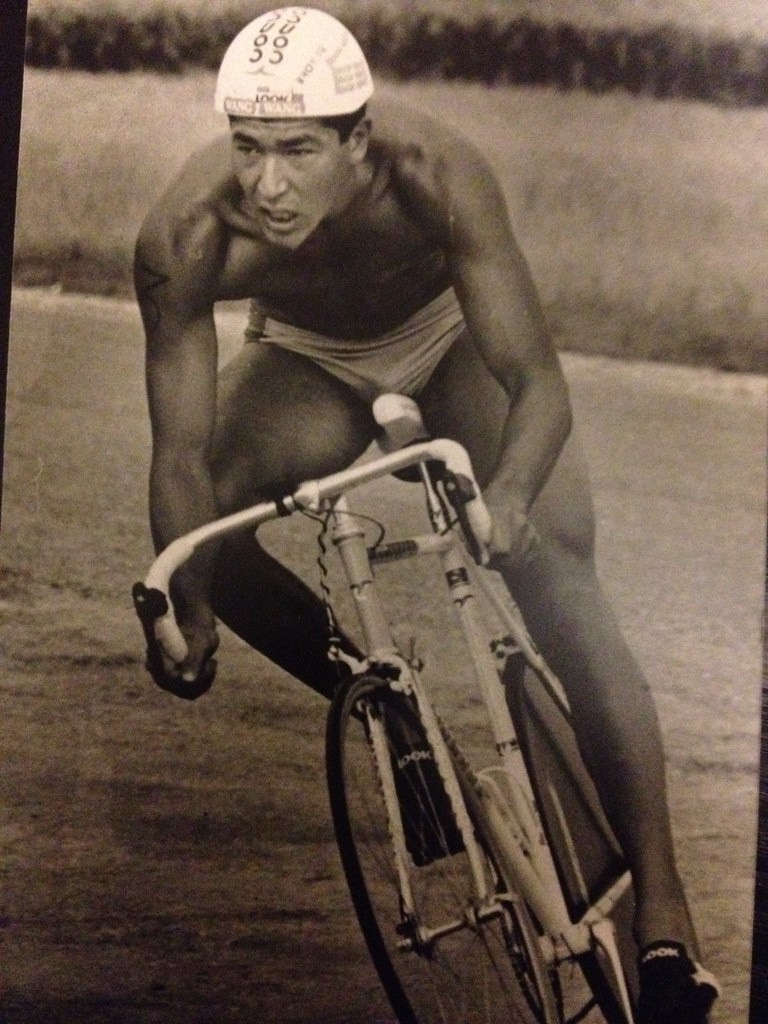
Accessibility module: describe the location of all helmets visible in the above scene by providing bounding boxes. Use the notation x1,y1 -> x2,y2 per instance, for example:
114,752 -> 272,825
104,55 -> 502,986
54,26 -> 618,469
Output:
215,7 -> 373,117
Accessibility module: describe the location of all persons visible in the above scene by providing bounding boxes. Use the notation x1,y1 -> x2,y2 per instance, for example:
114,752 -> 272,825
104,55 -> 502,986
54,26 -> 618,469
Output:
132,6 -> 721,1024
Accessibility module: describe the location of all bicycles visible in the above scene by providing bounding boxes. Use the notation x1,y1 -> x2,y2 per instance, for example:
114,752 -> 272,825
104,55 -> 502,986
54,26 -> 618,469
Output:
135,389 -> 716,1023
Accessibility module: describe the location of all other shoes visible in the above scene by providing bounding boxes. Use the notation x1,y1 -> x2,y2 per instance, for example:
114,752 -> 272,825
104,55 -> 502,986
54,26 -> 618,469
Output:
635,941 -> 721,1024
393,744 -> 464,864
